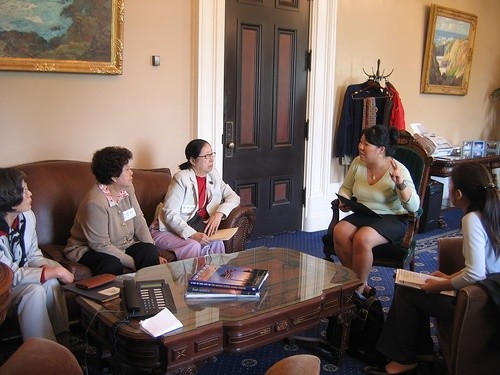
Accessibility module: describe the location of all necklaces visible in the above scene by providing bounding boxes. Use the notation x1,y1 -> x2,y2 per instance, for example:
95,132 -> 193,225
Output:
372,169 -> 381,180
197,190 -> 207,214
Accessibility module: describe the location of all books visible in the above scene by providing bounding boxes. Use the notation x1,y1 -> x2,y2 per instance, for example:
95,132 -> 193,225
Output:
183,263 -> 270,298
396,266 -> 455,296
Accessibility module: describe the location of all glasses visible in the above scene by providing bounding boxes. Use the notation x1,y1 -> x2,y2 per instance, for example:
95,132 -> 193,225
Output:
195,151 -> 217,159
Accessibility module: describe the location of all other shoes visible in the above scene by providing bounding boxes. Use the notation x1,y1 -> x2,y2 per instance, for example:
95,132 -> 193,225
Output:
355,285 -> 376,296
364,363 -> 427,375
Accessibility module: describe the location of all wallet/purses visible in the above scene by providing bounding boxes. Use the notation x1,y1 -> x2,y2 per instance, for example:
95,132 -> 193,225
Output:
75,273 -> 117,290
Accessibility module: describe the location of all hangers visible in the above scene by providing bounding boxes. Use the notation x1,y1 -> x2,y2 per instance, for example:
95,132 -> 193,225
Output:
351,73 -> 394,101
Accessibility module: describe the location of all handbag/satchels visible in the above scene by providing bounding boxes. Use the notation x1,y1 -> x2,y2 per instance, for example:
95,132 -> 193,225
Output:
326,296 -> 386,360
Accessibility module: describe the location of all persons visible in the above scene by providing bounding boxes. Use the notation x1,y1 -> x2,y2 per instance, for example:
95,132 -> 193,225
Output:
334,125 -> 422,295
372,161 -> 500,375
0,168 -> 79,349
65,139 -> 241,276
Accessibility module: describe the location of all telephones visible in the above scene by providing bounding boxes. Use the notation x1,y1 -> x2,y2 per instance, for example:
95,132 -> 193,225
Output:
120,278 -> 177,320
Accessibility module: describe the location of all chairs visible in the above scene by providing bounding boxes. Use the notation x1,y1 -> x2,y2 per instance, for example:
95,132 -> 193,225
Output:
322,146 -> 433,273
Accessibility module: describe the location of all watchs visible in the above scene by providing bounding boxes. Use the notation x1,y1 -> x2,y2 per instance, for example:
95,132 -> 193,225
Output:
397,180 -> 408,191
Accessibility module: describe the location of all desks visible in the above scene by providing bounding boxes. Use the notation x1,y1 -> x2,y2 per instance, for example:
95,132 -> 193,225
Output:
429,152 -> 500,179
76,248 -> 362,375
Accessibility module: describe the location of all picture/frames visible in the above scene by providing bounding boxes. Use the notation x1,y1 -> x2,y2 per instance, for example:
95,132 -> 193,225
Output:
419,4 -> 478,97
0,0 -> 124,75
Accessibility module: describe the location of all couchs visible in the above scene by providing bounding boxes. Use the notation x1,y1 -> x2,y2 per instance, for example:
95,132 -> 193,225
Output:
0,160 -> 257,342
427,235 -> 500,375
